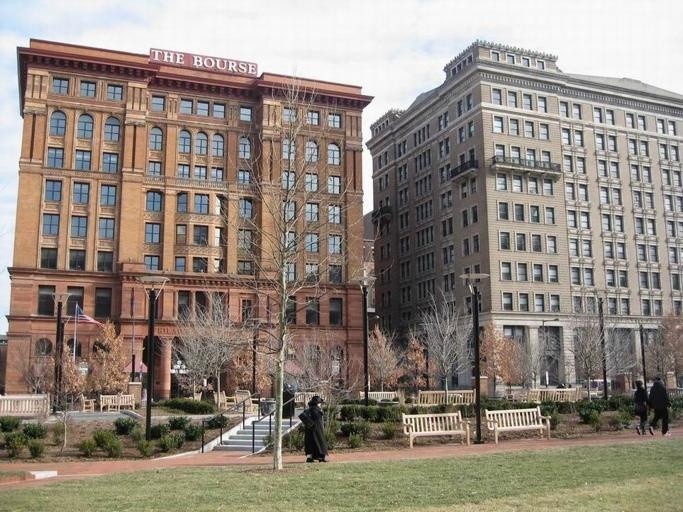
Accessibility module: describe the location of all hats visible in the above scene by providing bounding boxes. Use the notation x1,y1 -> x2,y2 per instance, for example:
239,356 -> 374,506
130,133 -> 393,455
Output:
635,380 -> 642,386
308,396 -> 322,404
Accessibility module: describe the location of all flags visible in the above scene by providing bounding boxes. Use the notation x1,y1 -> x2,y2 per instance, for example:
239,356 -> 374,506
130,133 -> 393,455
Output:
76,304 -> 103,327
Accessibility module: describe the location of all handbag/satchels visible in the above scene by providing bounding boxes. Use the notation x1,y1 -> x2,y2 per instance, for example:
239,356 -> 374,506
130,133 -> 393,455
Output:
298,409 -> 315,427
636,401 -> 646,412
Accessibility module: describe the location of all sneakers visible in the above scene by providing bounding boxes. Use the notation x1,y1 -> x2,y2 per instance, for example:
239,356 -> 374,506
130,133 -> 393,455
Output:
664,432 -> 671,437
649,426 -> 653,435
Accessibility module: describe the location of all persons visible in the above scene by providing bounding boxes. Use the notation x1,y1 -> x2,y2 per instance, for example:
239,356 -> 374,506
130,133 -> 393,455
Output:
649,376 -> 672,437
301,395 -> 332,462
633,380 -> 649,435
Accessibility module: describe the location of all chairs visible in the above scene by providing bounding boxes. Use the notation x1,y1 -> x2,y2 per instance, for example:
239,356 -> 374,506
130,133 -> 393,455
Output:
359,390 -> 476,407
402,412 -> 471,448
485,407 -> 551,445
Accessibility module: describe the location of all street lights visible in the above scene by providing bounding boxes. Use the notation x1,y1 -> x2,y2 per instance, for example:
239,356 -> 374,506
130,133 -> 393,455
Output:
351,277 -> 376,421
592,286 -> 610,399
51,293 -> 71,413
138,275 -> 169,441
460,273 -> 489,443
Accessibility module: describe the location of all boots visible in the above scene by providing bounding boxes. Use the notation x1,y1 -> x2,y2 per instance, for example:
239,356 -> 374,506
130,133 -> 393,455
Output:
636,426 -> 645,435
306,458 -> 329,462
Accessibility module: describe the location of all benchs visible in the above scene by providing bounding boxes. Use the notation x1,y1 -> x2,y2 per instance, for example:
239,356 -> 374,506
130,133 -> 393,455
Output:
0,393 -> 50,424
214,388 -> 324,412
79,391 -> 137,414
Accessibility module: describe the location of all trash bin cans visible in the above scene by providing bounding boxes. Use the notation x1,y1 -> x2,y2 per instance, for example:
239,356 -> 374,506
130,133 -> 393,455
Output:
260,398 -> 277,416
